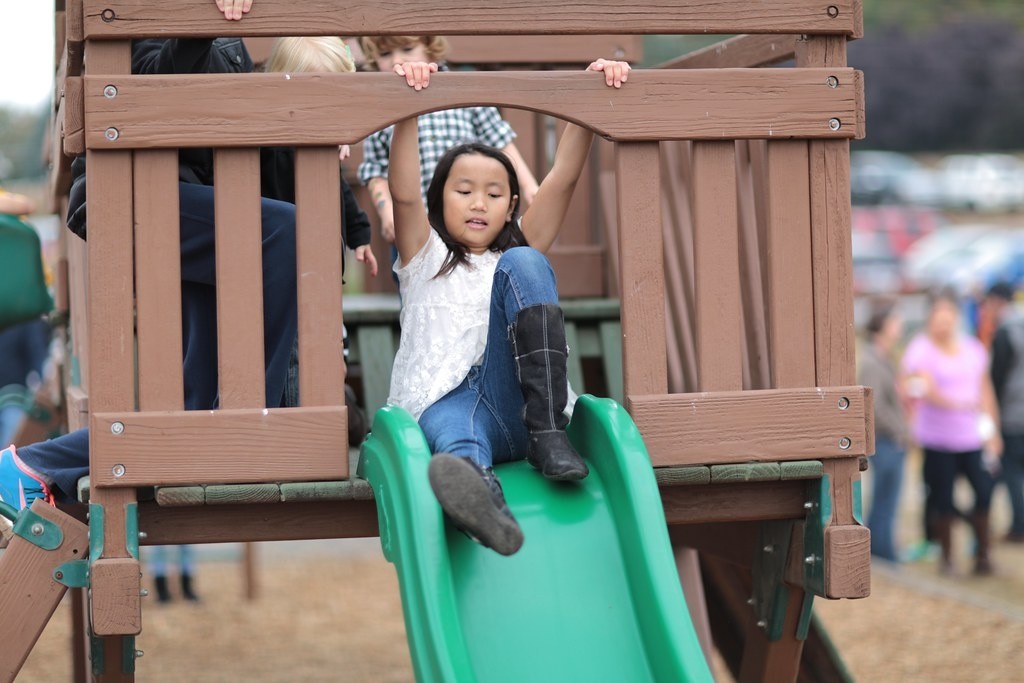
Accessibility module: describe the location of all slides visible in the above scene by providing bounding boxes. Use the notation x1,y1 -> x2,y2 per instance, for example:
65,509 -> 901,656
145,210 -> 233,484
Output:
363,396 -> 716,683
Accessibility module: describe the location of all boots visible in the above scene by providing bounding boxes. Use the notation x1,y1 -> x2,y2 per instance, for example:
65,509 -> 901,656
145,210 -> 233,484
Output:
969,515 -> 992,573
428,453 -> 524,556
508,304 -> 588,482
924,508 -> 950,571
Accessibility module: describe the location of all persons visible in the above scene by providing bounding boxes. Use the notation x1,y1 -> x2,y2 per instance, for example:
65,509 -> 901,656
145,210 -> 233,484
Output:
0,1 -> 541,527
386,57 -> 633,556
856,281 -> 1024,577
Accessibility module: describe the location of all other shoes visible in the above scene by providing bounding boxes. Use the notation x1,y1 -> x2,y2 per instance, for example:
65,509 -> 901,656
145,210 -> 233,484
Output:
344,384 -> 368,446
0,444 -> 56,527
1001,533 -> 1024,541
